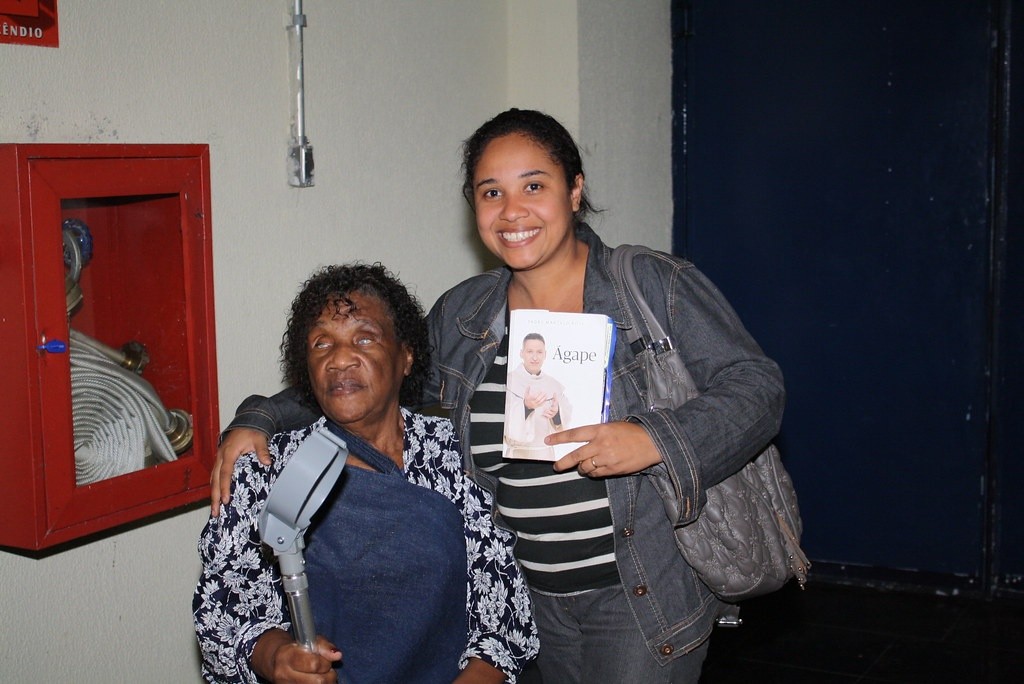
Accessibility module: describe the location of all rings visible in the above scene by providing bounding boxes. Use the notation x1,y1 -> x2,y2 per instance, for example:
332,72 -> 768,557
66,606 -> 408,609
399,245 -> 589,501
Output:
579,461 -> 584,471
590,458 -> 598,468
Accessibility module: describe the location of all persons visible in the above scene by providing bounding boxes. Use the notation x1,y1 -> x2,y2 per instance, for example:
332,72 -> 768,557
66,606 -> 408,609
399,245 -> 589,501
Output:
504,333 -> 572,461
210,107 -> 785,684
193,263 -> 541,684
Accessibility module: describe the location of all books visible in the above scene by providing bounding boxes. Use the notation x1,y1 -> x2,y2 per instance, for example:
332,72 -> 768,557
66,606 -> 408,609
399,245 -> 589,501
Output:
500,308 -> 610,463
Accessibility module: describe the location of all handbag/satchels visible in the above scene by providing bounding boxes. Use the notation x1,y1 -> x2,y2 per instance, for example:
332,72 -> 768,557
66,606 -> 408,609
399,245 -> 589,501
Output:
610,243 -> 811,603
